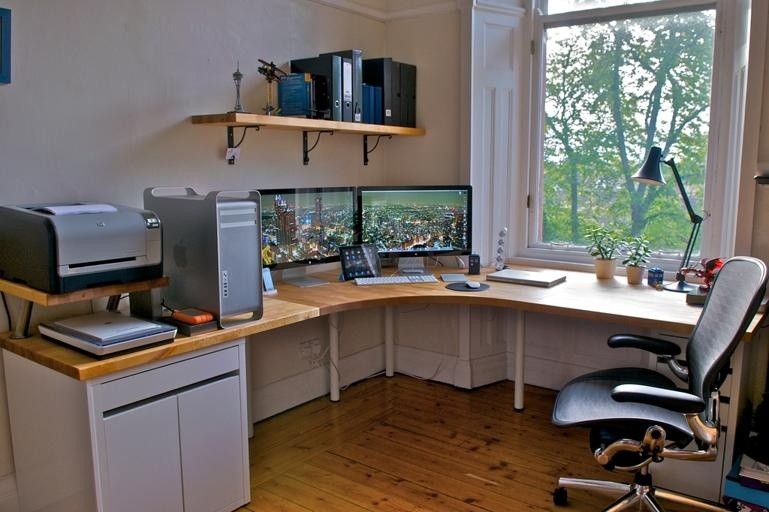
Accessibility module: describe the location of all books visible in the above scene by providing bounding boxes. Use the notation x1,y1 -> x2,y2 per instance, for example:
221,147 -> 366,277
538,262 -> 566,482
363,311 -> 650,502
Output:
738,429 -> 769,485
279,72 -> 317,118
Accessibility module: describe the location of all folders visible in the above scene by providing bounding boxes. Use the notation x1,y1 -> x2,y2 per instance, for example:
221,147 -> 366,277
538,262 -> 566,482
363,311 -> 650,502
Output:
290,50 -> 416,128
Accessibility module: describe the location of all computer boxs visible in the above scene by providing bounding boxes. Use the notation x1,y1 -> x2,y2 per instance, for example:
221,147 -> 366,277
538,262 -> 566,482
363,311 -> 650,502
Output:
143,186 -> 264,330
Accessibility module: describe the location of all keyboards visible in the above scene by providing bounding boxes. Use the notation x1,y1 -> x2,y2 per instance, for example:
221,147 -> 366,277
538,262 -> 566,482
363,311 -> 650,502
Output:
354,275 -> 438,286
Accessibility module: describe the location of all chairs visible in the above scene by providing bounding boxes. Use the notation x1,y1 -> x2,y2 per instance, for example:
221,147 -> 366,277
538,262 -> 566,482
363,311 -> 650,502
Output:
550,255 -> 769,511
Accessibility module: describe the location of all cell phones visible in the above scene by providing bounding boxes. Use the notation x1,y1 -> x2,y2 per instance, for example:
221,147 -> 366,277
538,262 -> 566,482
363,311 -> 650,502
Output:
262,268 -> 274,292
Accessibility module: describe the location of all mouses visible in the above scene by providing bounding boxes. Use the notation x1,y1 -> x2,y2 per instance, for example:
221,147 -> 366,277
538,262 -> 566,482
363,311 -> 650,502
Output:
464,280 -> 481,289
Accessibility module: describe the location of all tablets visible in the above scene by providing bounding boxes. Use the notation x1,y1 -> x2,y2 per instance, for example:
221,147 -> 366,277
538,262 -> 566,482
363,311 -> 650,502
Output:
339,245 -> 374,281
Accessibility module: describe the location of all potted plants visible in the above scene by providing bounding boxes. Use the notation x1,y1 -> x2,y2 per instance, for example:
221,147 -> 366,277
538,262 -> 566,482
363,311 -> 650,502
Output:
582,222 -> 629,279
621,232 -> 650,285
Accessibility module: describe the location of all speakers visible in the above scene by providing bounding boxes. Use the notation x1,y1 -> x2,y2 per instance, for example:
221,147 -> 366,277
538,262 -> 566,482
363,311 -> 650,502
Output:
495,225 -> 509,271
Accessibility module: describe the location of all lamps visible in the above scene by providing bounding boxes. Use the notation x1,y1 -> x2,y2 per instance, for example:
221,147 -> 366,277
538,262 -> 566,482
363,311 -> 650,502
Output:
631,145 -> 706,292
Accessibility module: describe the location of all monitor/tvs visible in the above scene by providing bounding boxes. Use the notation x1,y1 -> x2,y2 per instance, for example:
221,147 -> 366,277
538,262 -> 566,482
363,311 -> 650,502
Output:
257,186 -> 357,288
357,185 -> 472,275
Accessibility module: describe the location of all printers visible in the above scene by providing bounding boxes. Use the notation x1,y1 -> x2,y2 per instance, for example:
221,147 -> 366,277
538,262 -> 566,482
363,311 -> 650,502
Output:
0,200 -> 163,294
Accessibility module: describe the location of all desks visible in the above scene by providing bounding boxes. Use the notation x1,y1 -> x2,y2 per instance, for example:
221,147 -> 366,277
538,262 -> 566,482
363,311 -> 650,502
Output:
1,258 -> 765,512
